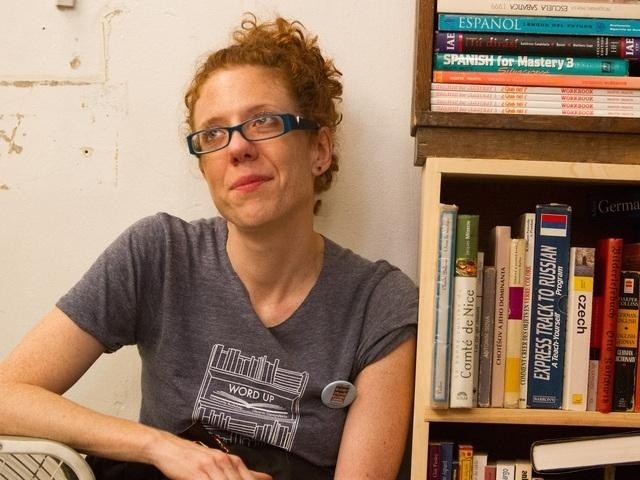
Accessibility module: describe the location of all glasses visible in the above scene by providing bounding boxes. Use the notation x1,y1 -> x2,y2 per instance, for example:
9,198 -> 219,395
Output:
186,112 -> 322,156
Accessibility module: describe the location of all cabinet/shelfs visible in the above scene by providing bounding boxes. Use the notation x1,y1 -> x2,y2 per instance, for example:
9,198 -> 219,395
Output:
409,0 -> 640,166
407,157 -> 640,480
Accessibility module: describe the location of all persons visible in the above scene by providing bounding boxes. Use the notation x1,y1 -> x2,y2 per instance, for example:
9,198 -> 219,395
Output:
1,12 -> 417,480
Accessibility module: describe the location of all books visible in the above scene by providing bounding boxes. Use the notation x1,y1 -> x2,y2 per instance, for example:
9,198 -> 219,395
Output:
432,71 -> 640,90
471,251 -> 485,410
436,1 -> 640,21
448,214 -> 480,411
614,269 -> 640,413
430,104 -> 640,118
430,90 -> 639,103
476,267 -> 497,408
526,205 -> 572,410
426,428 -> 640,480
433,53 -> 629,77
503,238 -> 526,409
433,32 -> 640,60
431,84 -> 640,95
430,205 -> 458,410
431,97 -> 639,111
517,213 -> 535,412
438,14 -> 640,38
562,246 -> 595,411
490,227 -> 511,409
587,262 -> 605,413
595,237 -> 623,414
